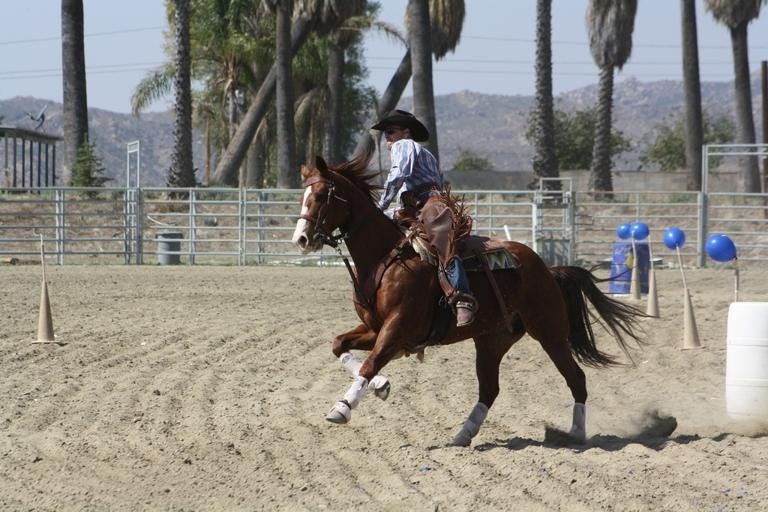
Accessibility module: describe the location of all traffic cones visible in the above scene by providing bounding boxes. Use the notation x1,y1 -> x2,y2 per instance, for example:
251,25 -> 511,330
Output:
630,258 -> 662,321
32,280 -> 58,345
679,288 -> 706,351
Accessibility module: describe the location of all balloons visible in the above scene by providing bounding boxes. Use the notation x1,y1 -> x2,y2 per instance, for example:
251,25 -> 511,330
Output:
616,222 -> 650,241
663,227 -> 685,250
704,233 -> 737,263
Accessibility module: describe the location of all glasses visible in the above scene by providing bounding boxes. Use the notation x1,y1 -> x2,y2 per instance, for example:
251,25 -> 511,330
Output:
383,126 -> 399,136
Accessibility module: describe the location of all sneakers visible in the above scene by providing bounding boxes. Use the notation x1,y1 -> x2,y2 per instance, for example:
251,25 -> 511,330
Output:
456,299 -> 480,328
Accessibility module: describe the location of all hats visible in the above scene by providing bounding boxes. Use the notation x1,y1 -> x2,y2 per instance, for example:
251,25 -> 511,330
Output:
370,109 -> 429,143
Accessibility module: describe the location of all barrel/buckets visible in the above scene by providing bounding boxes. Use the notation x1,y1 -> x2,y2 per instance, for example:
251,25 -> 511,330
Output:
609,243 -> 648,293
726,301 -> 768,438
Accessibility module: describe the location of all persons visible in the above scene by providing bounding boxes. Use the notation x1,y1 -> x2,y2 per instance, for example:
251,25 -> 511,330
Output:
370,109 -> 481,328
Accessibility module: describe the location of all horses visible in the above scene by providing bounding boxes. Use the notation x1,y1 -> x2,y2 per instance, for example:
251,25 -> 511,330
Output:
290,153 -> 675,448
